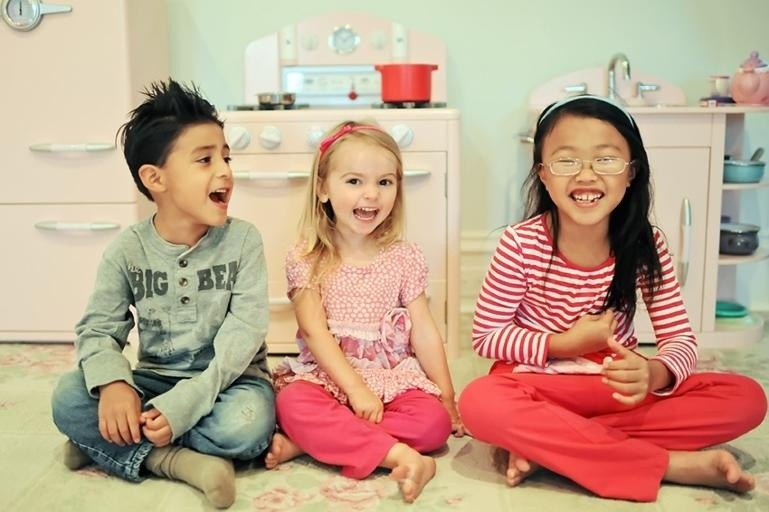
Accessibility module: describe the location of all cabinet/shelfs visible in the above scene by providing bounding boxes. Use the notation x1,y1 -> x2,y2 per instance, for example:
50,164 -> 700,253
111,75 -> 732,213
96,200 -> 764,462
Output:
626,106 -> 769,348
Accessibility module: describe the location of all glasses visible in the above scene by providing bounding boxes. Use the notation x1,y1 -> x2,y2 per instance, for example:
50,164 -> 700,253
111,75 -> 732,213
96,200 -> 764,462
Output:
538,156 -> 637,176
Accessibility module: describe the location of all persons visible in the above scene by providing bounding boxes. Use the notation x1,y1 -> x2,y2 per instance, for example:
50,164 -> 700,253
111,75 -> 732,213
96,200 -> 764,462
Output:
51,76 -> 277,509
458,93 -> 769,497
262,119 -> 476,507
